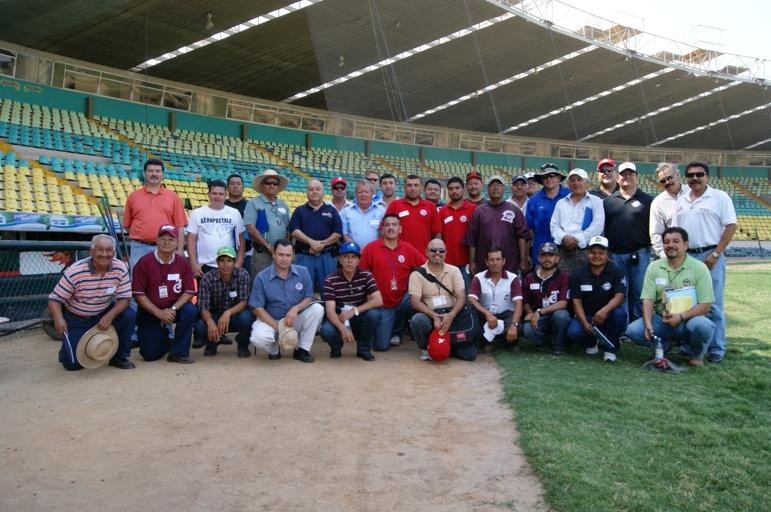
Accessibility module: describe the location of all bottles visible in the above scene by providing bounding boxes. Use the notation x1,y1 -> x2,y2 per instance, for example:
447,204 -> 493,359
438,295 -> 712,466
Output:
340,307 -> 352,332
655,337 -> 663,359
166,306 -> 176,343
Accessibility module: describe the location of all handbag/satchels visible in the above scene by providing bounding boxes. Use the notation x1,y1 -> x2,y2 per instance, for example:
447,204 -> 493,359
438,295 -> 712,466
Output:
431,302 -> 482,341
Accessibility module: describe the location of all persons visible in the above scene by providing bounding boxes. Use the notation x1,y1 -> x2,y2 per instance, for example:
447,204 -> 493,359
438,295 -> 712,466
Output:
123,158 -> 383,362
524,158 -> 654,362
648,162 -> 737,362
49,234 -> 136,369
625,227 -> 715,369
358,170 -> 539,361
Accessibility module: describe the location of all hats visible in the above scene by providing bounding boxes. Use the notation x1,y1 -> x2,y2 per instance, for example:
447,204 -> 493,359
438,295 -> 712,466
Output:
523,172 -> 537,183
486,175 -> 506,186
215,245 -> 237,261
157,224 -> 178,240
536,242 -> 560,256
596,158 -> 617,174
330,177 -> 347,188
510,174 -> 527,184
425,326 -> 452,362
73,320 -> 119,370
586,236 -> 608,252
566,167 -> 588,181
482,318 -> 506,343
252,168 -> 289,194
533,163 -> 566,184
338,242 -> 361,259
615,162 -> 637,175
464,171 -> 483,182
275,316 -> 300,352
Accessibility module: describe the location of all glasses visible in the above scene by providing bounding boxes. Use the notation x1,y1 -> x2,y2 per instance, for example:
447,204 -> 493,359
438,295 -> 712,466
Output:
659,175 -> 674,183
599,167 -> 612,174
541,173 -> 554,181
263,178 -> 279,186
333,186 -> 345,191
429,248 -> 445,255
366,176 -> 377,181
685,173 -> 704,177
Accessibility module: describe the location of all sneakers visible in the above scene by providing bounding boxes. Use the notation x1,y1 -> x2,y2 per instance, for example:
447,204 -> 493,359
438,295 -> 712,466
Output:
167,351 -> 192,363
205,338 -> 219,357
292,348 -> 314,365
602,350 -> 618,364
268,344 -> 282,360
189,336 -> 205,350
218,332 -> 232,345
108,354 -> 134,370
708,352 -> 722,363
583,338 -> 601,358
330,344 -> 343,359
235,343 -> 251,358
357,349 -> 375,363
418,349 -> 432,361
689,356 -> 705,367
